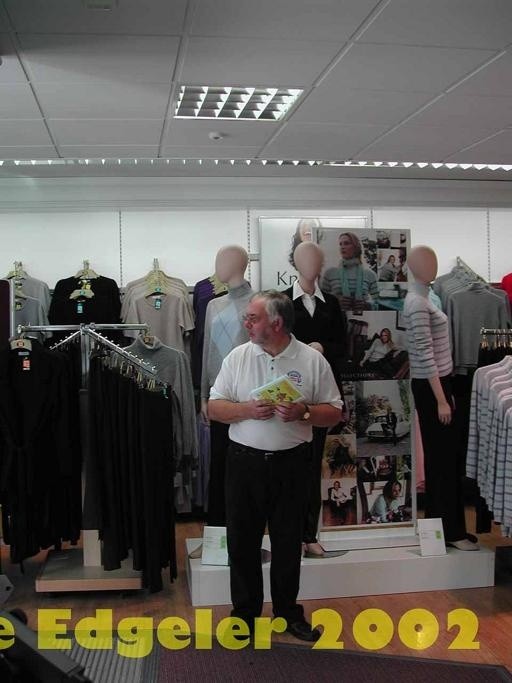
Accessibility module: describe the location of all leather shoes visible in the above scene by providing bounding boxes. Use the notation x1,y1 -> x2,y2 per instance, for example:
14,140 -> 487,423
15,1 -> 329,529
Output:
274,616 -> 321,641
231,609 -> 260,640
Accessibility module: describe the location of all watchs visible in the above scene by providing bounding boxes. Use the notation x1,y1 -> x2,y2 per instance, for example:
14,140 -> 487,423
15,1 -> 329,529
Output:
298,402 -> 311,422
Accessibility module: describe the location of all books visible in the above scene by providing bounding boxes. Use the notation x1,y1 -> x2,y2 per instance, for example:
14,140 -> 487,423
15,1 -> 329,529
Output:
250,374 -> 306,408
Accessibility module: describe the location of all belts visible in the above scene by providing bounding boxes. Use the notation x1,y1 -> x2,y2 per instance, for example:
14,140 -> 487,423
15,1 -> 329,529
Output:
231,442 -> 312,458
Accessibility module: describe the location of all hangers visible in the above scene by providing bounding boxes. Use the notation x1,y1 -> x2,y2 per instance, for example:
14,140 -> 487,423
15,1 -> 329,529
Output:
7,260 -> 27,300
146,263 -> 169,296
68,261 -> 98,301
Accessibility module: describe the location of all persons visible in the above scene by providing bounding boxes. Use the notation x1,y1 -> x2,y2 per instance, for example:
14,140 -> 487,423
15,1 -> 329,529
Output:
326,437 -> 411,523
375,406 -> 397,447
279,240 -> 349,559
359,327 -> 405,367
188,245 -> 256,561
204,289 -> 344,642
402,246 -> 480,552
289,218 -> 324,270
380,253 -> 402,282
322,232 -> 380,311
